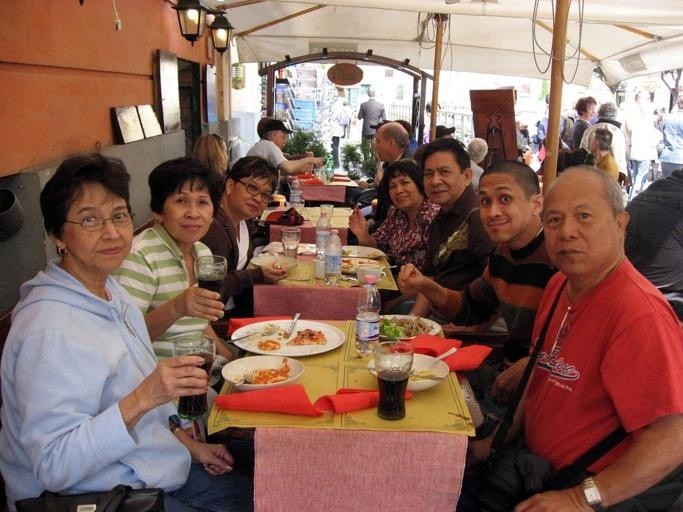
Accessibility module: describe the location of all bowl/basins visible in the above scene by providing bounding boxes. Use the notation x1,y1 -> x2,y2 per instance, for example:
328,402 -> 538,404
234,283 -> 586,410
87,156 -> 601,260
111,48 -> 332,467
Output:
368,352 -> 450,392
222,356 -> 305,391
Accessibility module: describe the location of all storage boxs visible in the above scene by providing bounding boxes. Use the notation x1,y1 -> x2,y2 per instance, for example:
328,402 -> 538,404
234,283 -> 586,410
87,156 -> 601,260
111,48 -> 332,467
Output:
286,77 -> 321,99
290,66 -> 317,78
287,109 -> 314,131
288,98 -> 316,121
288,88 -> 320,110
287,69 -> 318,88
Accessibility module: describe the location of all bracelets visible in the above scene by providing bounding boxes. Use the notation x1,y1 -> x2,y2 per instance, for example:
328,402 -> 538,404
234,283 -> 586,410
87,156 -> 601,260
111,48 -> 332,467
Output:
173,426 -> 183,434
364,236 -> 375,245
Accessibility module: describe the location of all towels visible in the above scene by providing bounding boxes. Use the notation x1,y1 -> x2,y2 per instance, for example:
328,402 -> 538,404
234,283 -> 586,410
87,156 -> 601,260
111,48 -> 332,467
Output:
314,388 -> 413,414
225,316 -> 292,336
215,383 -> 325,417
389,334 -> 463,358
441,344 -> 493,369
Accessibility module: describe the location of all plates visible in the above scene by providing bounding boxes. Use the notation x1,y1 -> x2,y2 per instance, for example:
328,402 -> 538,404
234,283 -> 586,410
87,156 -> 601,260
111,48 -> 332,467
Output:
379,315 -> 443,340
250,256 -> 298,271
341,246 -> 386,258
341,258 -> 384,274
231,320 -> 347,357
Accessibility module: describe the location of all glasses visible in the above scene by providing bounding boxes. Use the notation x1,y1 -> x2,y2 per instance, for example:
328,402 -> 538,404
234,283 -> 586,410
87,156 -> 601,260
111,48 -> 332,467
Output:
63,211 -> 135,232
232,178 -> 273,203
212,133 -> 221,144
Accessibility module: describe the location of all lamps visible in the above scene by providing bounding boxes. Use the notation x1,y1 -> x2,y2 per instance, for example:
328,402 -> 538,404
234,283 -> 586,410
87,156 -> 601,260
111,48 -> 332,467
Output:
171,0 -> 212,47
207,9 -> 235,55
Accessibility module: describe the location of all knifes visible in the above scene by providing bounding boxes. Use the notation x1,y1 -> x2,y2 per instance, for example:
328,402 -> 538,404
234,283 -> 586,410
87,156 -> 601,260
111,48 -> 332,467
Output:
284,313 -> 301,339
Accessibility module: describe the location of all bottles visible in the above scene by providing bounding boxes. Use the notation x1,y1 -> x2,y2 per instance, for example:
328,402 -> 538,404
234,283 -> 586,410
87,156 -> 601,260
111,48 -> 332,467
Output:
354,275 -> 380,355
289,177 -> 300,210
320,165 -> 328,183
314,166 -> 321,182
326,156 -> 331,164
324,229 -> 341,286
326,158 -> 333,178
315,212 -> 331,260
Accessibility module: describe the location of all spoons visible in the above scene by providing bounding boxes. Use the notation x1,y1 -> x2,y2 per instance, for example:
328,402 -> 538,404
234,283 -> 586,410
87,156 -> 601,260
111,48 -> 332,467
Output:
225,325 -> 280,345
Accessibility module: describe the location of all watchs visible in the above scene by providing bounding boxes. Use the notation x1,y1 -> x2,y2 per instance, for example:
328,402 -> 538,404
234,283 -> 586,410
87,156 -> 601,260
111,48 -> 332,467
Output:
168,414 -> 182,430
583,476 -> 606,512
369,183 -> 373,187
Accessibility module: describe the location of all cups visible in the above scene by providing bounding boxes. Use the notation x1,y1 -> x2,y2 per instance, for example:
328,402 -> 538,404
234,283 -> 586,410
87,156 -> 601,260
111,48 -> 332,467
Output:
280,228 -> 301,259
195,256 -> 227,306
312,258 -> 325,280
357,264 -> 386,284
376,342 -> 413,422
320,204 -> 334,226
174,335 -> 214,421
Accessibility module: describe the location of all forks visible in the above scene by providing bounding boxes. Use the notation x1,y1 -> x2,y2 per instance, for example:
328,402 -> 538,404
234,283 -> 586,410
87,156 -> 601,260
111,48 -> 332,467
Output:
234,374 -> 251,387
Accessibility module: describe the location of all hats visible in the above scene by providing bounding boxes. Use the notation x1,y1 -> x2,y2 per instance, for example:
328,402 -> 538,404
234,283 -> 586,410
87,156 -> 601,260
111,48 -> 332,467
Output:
370,120 -> 392,130
257,118 -> 293,134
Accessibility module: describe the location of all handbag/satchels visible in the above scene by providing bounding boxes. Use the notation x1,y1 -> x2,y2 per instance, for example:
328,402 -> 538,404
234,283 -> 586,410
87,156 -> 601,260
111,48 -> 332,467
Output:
16,484 -> 165,512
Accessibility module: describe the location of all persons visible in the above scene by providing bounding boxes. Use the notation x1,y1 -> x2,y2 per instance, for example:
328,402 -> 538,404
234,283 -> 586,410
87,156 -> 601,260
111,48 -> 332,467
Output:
246,119 -> 323,195
1,153 -> 255,512
623,169 -> 682,322
423,102 -> 441,144
467,137 -> 489,195
394,120 -> 419,159
341,101 -> 352,139
192,134 -> 229,180
397,160 -> 559,440
347,122 -> 417,245
380,138 -> 498,323
351,120 -> 392,208
358,87 -> 386,161
108,157 -> 245,427
456,166 -> 683,512
198,156 -> 288,338
349,161 -> 443,297
516,92 -> 683,173
